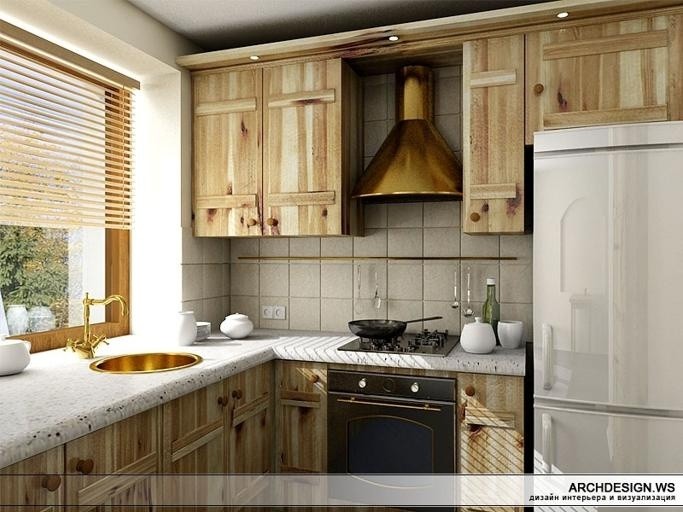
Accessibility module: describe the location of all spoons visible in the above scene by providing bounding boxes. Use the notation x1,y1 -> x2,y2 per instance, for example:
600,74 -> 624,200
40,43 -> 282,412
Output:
451,271 -> 458,309
463,267 -> 475,318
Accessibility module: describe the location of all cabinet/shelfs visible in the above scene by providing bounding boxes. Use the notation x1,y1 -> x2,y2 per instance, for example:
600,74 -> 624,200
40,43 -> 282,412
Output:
0,405 -> 162,512
162,360 -> 275,512
456,373 -> 524,512
461,33 -> 525,234
275,359 -> 329,512
190,57 -> 365,237
525,11 -> 682,146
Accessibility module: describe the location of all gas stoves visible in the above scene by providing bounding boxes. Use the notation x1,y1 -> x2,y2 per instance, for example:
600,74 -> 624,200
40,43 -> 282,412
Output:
337,330 -> 459,358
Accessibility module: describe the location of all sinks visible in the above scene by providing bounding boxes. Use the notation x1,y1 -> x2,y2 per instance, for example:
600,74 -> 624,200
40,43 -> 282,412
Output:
89,351 -> 204,374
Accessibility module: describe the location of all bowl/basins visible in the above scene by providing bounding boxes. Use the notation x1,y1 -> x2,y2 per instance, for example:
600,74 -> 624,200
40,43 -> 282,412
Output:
196,321 -> 211,342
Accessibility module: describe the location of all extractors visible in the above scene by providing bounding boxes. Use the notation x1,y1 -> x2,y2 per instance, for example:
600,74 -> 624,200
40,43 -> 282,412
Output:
351,64 -> 463,205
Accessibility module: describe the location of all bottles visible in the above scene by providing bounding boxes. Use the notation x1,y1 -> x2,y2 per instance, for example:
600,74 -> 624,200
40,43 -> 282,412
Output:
219,313 -> 254,339
178,311 -> 197,347
481,277 -> 500,346
7,304 -> 28,334
27,304 -> 56,331
460,317 -> 496,354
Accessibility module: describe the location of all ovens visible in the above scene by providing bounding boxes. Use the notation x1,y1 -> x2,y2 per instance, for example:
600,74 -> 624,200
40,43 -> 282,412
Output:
325,368 -> 457,512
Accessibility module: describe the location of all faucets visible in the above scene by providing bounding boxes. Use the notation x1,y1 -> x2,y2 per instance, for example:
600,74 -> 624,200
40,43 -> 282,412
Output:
61,291 -> 128,360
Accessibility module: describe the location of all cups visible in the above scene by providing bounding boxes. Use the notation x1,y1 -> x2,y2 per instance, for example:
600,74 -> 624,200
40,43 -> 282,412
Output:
497,319 -> 523,348
0,334 -> 31,377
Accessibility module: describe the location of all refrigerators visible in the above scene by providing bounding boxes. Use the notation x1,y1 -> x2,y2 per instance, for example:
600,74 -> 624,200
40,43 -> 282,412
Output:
531,120 -> 683,512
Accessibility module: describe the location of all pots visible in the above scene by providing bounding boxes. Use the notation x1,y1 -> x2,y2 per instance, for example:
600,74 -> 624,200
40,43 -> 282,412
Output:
346,315 -> 443,337
